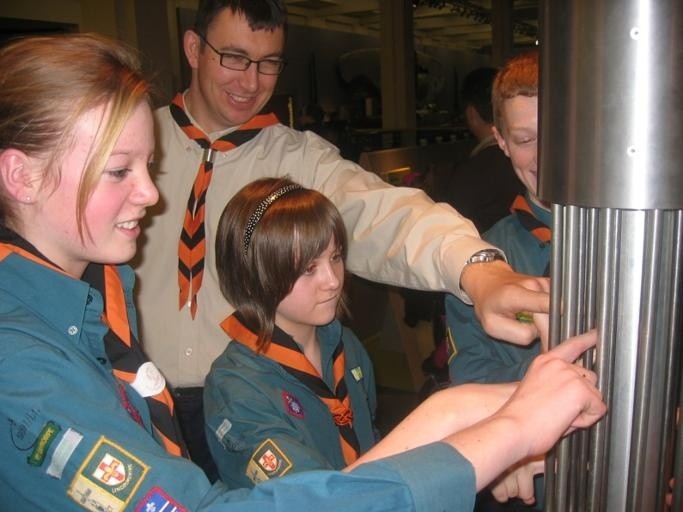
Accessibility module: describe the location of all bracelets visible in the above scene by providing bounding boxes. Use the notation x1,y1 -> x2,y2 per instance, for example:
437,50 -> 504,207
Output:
464,249 -> 501,267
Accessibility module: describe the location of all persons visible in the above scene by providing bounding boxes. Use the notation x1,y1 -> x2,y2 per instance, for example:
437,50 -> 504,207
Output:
443,47 -> 552,512
129,1 -> 550,488
439,67 -> 529,237
203,172 -> 547,507
1,31 -> 609,512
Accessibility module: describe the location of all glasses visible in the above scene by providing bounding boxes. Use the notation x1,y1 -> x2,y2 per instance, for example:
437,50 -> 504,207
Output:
204,39 -> 289,77
